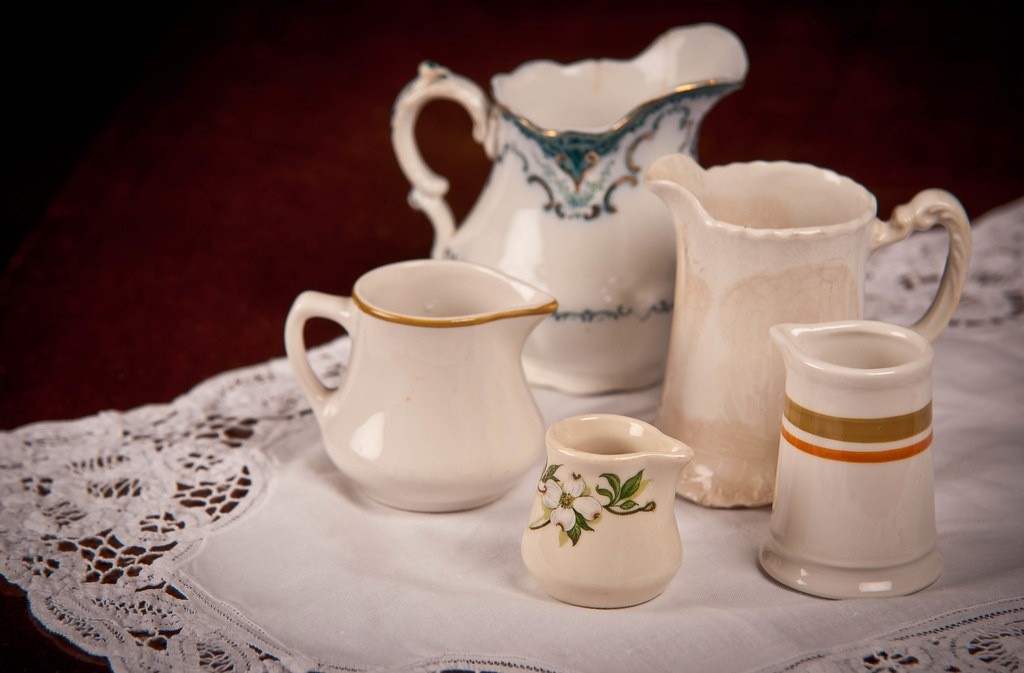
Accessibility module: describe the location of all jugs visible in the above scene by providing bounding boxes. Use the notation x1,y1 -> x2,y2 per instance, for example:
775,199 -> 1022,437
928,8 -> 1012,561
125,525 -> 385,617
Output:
390,24 -> 749,397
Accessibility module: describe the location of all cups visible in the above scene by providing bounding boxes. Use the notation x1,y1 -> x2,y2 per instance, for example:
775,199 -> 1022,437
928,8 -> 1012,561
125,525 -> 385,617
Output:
645,150 -> 972,508
520,412 -> 694,610
283,259 -> 558,513
757,321 -> 945,600
